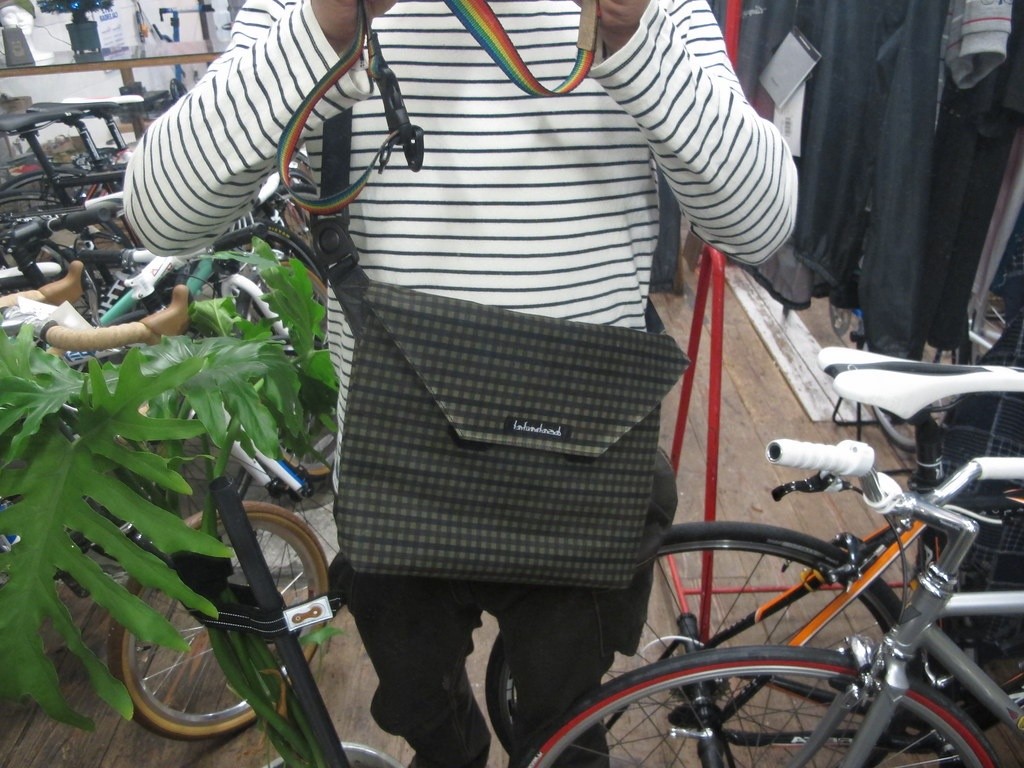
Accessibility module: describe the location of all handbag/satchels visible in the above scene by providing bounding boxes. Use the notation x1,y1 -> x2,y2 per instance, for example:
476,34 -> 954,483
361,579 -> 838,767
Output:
326,282 -> 692,600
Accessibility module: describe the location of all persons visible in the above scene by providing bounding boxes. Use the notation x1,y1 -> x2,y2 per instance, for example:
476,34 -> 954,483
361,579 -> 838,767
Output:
118,0 -> 803,768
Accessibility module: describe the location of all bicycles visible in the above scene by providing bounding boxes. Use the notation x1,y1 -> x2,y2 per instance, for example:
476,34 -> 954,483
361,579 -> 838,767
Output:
14,75 -> 1024,768
